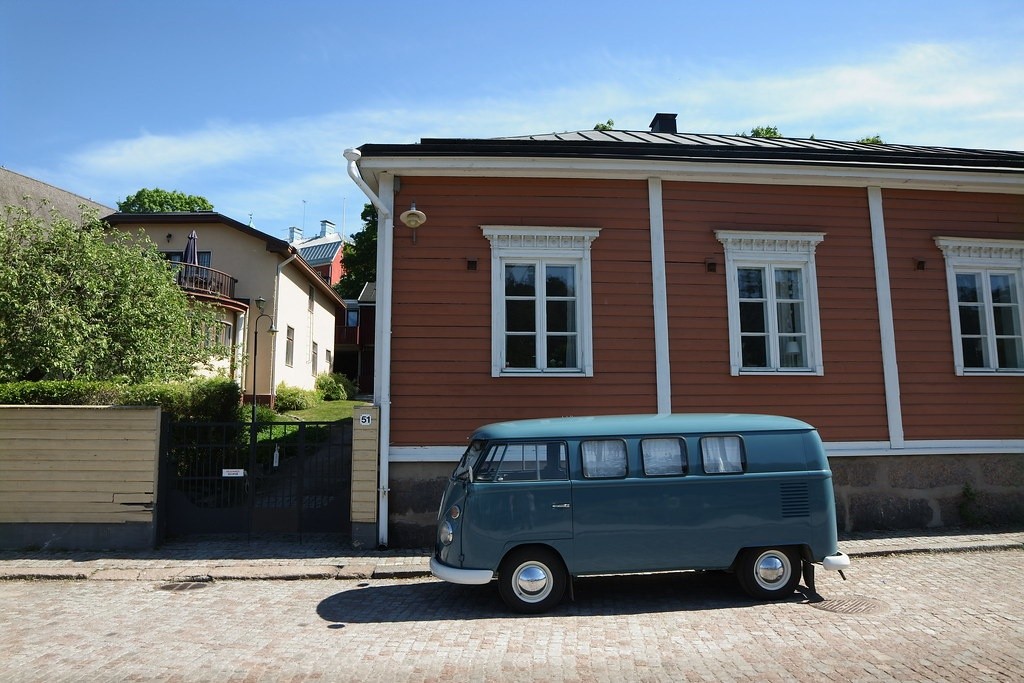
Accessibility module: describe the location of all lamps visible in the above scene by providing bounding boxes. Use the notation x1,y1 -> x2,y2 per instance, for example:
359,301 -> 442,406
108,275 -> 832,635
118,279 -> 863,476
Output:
399,201 -> 427,245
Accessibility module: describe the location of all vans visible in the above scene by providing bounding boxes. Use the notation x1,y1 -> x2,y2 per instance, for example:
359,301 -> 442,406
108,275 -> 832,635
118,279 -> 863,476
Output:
428,412 -> 849,613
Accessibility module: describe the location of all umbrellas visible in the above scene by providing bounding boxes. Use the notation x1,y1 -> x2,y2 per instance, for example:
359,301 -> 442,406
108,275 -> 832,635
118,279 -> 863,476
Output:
182,229 -> 199,274
